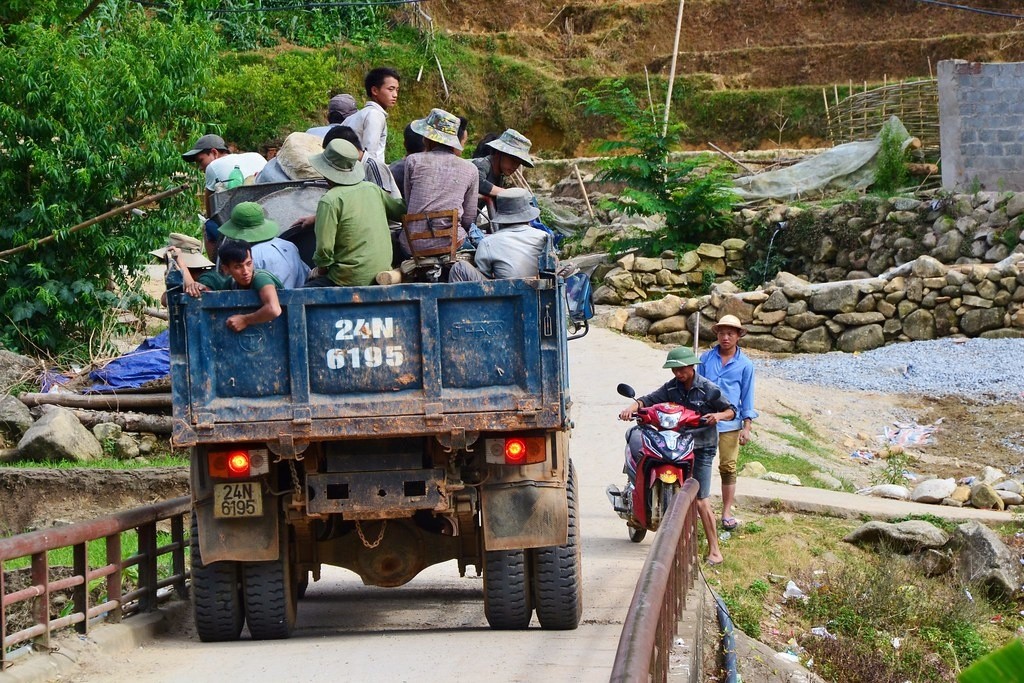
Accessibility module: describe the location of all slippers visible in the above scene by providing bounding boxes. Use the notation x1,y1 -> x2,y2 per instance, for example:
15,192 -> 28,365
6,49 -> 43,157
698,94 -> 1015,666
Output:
722,517 -> 737,528
703,558 -> 722,566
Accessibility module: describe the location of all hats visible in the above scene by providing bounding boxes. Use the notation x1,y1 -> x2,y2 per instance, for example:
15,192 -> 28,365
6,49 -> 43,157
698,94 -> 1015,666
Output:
410,108 -> 464,151
485,128 -> 535,169
663,347 -> 702,368
711,315 -> 748,338
489,188 -> 540,223
328,94 -> 359,118
217,202 -> 279,242
182,134 -> 228,163
308,138 -> 366,185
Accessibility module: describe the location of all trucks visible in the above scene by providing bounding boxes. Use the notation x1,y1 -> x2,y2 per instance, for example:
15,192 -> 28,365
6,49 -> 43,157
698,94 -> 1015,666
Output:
161,188 -> 597,643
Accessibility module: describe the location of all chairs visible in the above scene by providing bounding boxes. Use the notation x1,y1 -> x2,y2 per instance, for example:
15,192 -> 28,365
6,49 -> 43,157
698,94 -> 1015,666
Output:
401,207 -> 474,282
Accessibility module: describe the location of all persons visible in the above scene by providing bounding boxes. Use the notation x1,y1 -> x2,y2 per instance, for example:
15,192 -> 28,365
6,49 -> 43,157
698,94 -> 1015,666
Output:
620,346 -> 737,567
148,66 -> 560,332
696,314 -> 758,529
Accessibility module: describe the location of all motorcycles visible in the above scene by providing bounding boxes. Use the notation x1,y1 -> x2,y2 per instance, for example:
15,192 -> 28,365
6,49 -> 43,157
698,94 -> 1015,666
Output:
605,382 -> 721,543
